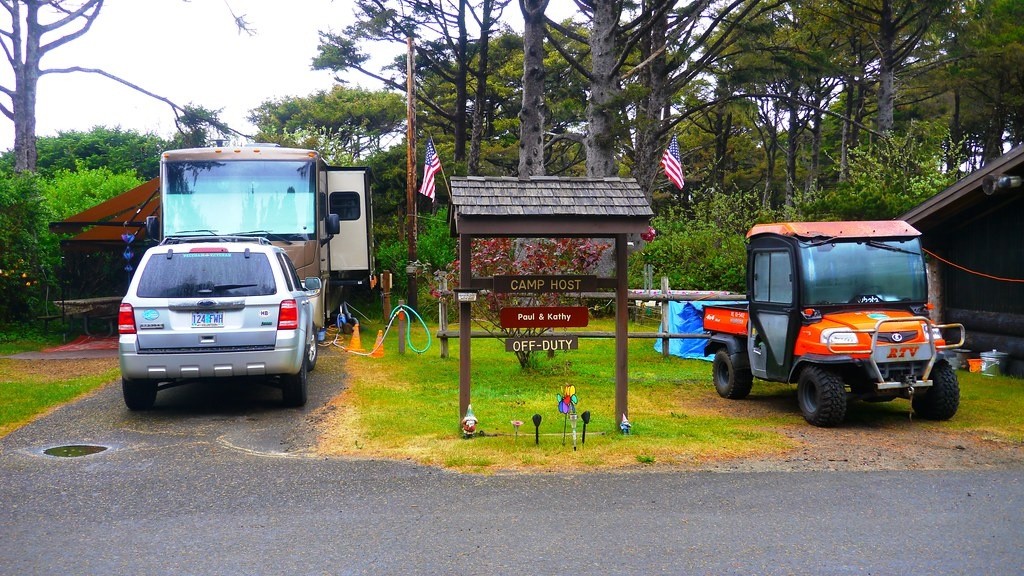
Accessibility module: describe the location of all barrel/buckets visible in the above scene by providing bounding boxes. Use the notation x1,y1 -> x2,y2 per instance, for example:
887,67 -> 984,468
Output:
981,350 -> 1009,378
953,349 -> 972,366
967,359 -> 983,374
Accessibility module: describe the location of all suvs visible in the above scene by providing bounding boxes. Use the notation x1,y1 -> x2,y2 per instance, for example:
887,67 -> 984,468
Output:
117,235 -> 322,411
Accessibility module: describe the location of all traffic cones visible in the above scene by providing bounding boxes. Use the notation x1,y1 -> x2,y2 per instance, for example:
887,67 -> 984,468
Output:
347,322 -> 366,352
370,330 -> 386,359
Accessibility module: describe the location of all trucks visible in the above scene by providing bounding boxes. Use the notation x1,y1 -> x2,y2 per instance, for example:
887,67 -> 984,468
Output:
145,144 -> 372,331
702,221 -> 968,427
